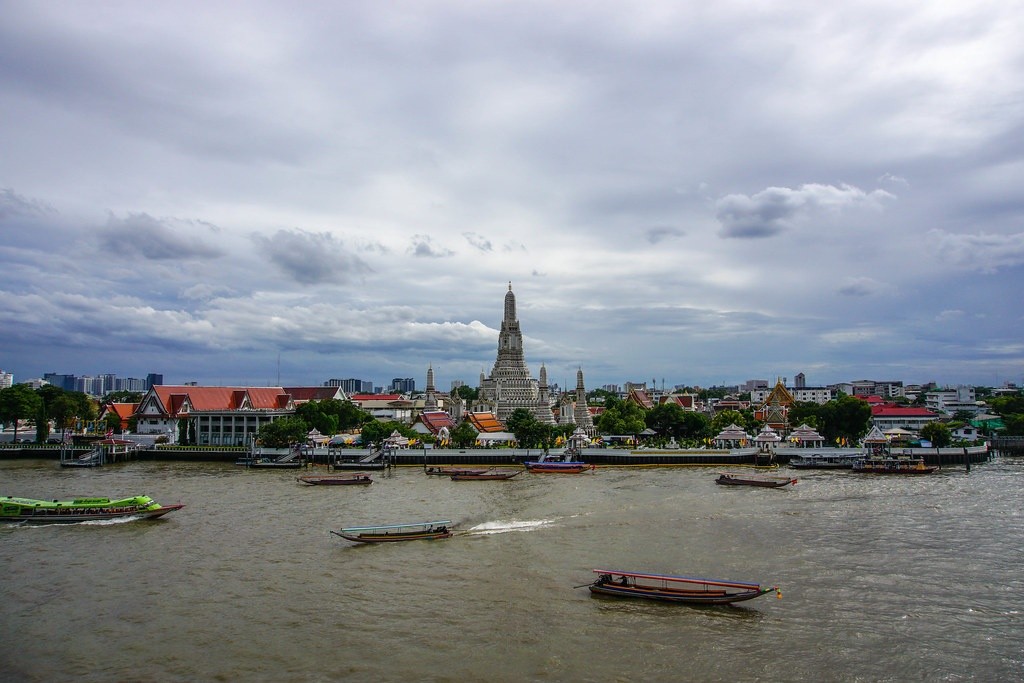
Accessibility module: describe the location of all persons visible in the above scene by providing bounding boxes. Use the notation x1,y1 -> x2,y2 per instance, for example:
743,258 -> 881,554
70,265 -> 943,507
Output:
619,576 -> 627,584
429,525 -> 433,532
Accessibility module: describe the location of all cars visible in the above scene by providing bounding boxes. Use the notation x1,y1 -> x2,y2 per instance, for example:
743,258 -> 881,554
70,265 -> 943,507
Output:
10,437 -> 61,445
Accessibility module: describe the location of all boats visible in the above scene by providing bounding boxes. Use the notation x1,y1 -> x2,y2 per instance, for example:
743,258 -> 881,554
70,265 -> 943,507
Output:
295,472 -> 375,485
851,448 -> 938,475
424,466 -> 522,481
788,453 -> 856,470
0,495 -> 187,523
521,446 -> 592,473
713,472 -> 798,488
329,521 -> 453,544
587,567 -> 781,606
